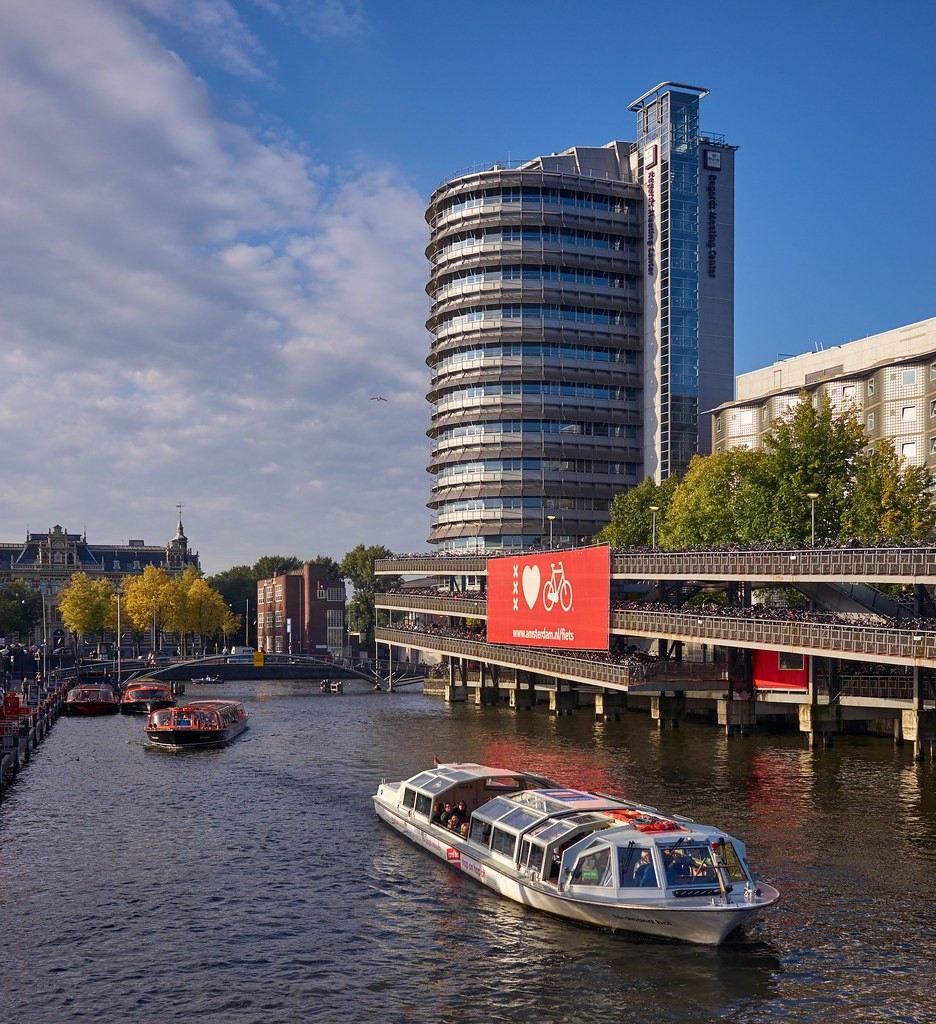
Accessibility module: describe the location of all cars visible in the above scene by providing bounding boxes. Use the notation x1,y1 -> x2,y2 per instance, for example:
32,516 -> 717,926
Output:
53,647 -> 87,658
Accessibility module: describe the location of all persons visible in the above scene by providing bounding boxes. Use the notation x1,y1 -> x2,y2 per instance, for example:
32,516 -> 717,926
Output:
148,650 -> 158,669
35,672 -> 43,690
429,798 -> 685,889
404,656 -> 409,663
222,646 -> 230,665
259,647 -> 266,665
21,678 -> 31,704
419,659 -> 426,665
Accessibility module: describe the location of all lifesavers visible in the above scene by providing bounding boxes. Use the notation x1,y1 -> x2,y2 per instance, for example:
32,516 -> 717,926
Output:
630,818 -> 676,830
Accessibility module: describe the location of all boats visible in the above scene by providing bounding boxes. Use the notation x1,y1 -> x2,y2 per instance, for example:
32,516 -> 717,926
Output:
120,676 -> 179,716
143,699 -> 251,750
191,678 -> 224,685
62,683 -> 120,715
371,750 -> 782,950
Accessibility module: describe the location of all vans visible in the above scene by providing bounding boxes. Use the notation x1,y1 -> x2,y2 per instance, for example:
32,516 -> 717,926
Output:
231,647 -> 256,655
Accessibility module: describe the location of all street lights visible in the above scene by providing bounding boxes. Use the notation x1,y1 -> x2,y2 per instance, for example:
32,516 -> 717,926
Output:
21,593 -> 48,678
229,598 -> 249,647
547,516 -> 556,550
0,642 -> 25,681
650,506 -> 659,550
807,493 -> 820,547
